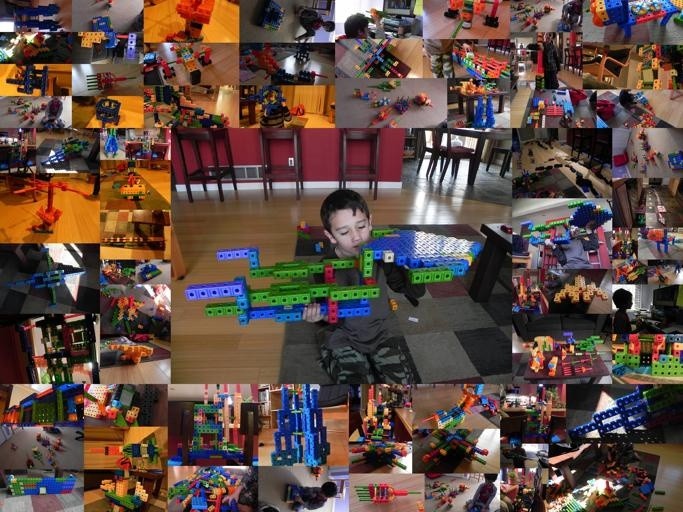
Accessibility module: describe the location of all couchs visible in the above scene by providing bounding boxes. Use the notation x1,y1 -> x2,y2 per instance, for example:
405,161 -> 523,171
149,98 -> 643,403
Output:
513,300 -> 608,342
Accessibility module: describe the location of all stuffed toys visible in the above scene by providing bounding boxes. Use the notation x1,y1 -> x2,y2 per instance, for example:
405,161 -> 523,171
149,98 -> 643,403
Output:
0,0 -> 683,512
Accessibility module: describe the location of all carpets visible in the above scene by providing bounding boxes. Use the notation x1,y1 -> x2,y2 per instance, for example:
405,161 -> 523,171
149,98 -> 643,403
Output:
84,497 -> 166,512
513,168 -> 587,198
0,243 -> 99,314
520,89 -> 596,128
413,429 -> 484,473
100,170 -> 171,210
100,210 -> 165,251
280,223 -> 513,382
550,448 -> 660,511
597,90 -> 676,128
467,390 -> 500,426
36,138 -> 100,174
101,338 -> 171,368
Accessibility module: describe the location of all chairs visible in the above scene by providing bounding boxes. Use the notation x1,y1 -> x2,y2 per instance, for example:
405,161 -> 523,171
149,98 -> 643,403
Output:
105,33 -> 118,60
175,128 -> 238,204
0,147 -> 36,173
500,414 -> 528,443
415,129 -> 513,186
582,48 -> 632,82
571,128 -> 612,171
258,128 -> 302,201
338,126 -> 381,201
564,46 -> 581,77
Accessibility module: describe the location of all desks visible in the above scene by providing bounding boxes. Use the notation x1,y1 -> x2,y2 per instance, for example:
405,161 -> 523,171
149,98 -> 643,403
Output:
632,312 -> 683,334
454,90 -> 507,126
524,349 -> 609,385
129,470 -> 166,511
366,14 -> 423,40
512,256 -> 531,268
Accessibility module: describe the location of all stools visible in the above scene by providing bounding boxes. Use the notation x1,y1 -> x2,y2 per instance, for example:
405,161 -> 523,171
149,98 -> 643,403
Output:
469,223 -> 514,303
